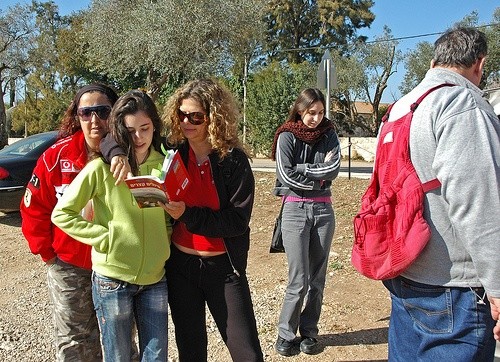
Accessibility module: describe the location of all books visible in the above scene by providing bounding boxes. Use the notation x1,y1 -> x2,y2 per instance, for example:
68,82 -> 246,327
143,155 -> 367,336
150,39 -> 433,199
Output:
124,150 -> 192,209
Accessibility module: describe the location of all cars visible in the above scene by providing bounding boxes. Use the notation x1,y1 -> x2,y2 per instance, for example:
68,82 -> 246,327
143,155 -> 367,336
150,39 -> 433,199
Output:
0,128 -> 70,216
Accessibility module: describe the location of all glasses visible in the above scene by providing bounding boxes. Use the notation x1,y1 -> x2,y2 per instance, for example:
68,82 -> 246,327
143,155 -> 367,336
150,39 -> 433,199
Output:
176,108 -> 207,126
77,104 -> 112,121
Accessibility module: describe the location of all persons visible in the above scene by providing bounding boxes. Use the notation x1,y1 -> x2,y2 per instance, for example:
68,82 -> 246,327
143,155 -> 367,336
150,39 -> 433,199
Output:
51,91 -> 173,362
271,88 -> 341,356
373,26 -> 500,362
99,79 -> 264,362
20,83 -> 119,362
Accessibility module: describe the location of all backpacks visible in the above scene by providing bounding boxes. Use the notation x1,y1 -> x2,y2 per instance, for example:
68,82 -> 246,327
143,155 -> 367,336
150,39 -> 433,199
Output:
351,81 -> 469,281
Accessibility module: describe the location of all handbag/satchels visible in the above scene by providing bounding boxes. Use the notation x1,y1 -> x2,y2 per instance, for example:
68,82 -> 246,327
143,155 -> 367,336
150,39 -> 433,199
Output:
268,217 -> 285,255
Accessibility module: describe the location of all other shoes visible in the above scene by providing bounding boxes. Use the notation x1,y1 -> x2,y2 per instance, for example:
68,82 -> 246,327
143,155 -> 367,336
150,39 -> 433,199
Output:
299,336 -> 317,354
276,335 -> 292,356
